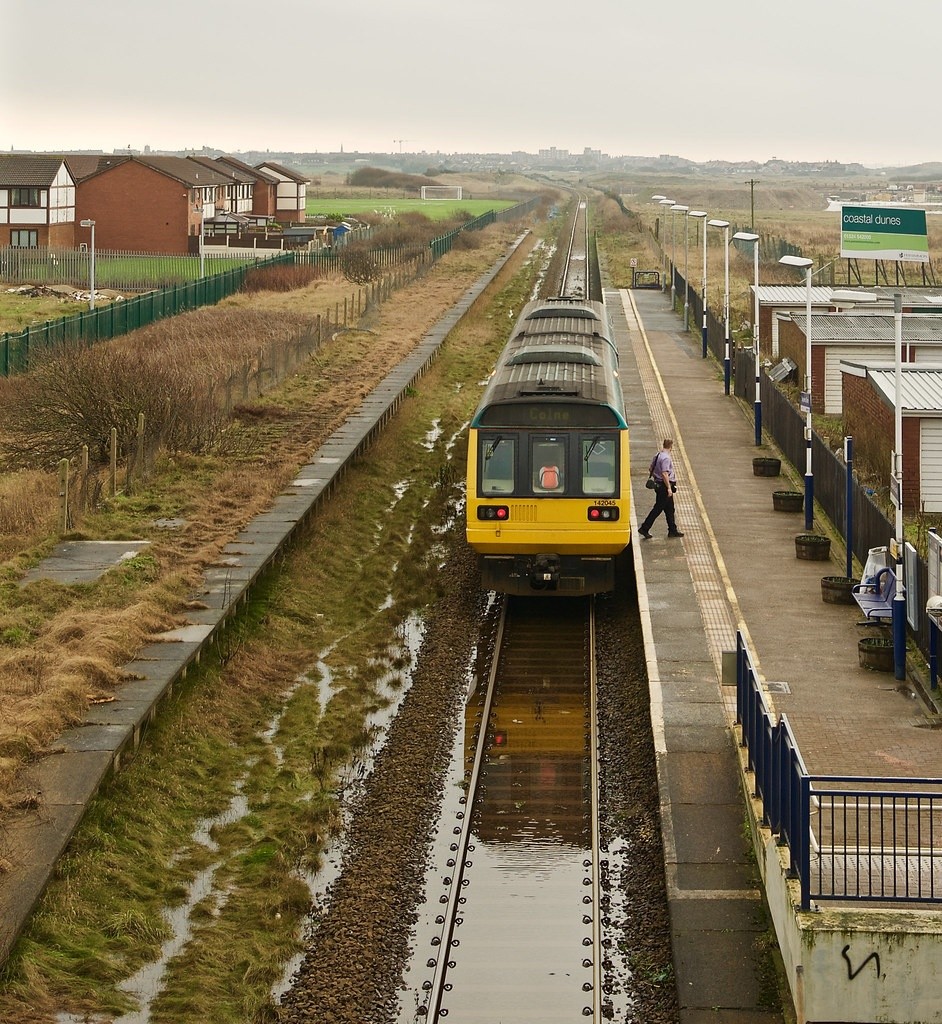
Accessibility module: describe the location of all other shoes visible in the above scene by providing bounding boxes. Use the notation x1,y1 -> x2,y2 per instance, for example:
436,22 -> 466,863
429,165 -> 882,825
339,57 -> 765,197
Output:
668,531 -> 684,537
638,527 -> 653,539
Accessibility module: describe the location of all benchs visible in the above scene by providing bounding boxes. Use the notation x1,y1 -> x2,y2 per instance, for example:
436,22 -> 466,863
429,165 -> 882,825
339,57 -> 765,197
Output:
851,567 -> 907,627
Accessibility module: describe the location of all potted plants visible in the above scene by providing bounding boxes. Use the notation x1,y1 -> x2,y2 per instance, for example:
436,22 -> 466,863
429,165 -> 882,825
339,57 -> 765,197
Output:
753,455 -> 781,477
795,534 -> 832,560
772,490 -> 804,512
856,636 -> 896,672
822,576 -> 861,605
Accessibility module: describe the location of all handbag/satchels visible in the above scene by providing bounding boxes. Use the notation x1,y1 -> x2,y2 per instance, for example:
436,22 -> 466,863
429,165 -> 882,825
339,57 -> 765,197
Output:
646,480 -> 655,489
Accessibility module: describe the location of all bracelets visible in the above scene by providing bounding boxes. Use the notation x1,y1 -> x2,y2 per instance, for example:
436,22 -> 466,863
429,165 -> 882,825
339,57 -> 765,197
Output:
668,485 -> 671,489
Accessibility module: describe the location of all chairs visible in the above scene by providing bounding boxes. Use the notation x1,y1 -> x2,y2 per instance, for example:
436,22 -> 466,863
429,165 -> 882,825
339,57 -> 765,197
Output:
488,446 -> 552,479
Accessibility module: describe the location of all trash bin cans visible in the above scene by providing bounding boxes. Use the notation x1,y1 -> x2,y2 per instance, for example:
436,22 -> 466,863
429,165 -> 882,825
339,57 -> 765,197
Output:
857,546 -> 888,596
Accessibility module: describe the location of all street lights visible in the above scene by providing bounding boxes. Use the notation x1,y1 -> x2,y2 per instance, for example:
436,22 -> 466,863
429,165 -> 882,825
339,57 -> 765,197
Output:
733,231 -> 762,445
708,219 -> 733,396
192,208 -> 204,280
651,193 -> 667,295
779,254 -> 815,532
79,218 -> 96,312
688,210 -> 708,358
661,199 -> 677,311
671,204 -> 690,334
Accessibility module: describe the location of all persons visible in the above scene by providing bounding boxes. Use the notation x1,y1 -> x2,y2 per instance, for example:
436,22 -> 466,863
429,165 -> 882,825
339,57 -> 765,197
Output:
637,438 -> 684,538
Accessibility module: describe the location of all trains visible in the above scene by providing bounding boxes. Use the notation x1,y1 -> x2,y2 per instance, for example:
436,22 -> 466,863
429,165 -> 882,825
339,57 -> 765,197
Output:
462,296 -> 633,602
